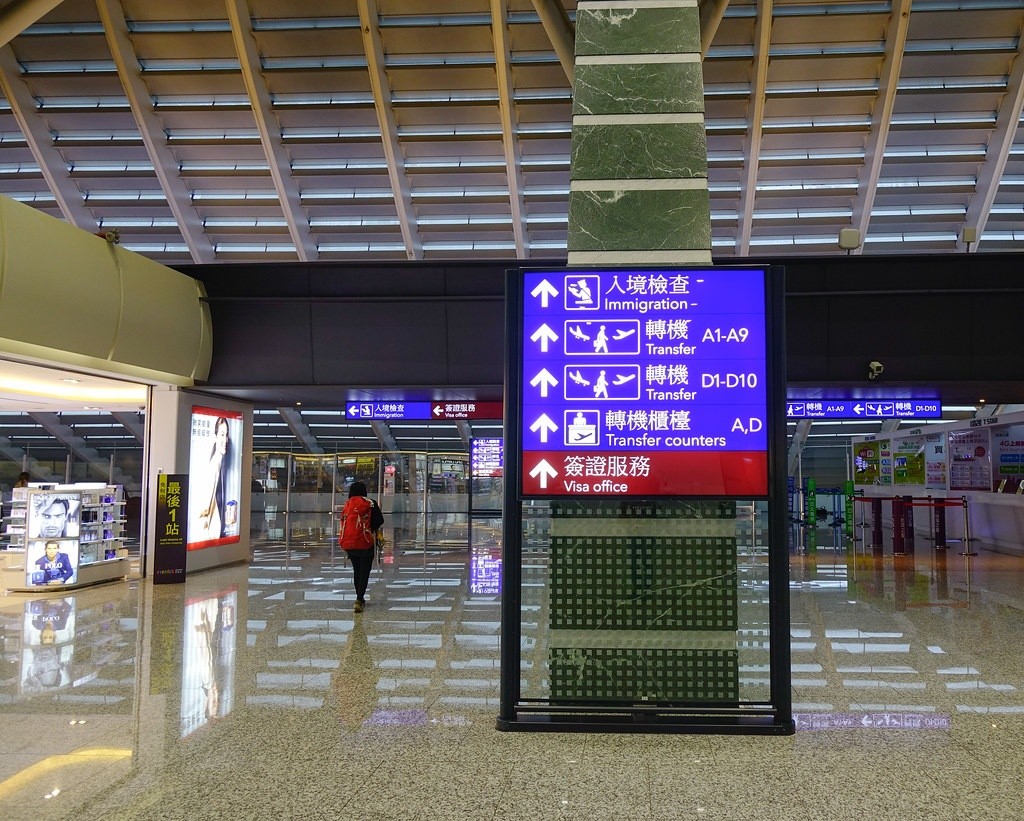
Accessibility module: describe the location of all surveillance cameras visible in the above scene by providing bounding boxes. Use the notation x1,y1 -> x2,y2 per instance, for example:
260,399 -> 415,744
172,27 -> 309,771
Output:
868,361 -> 884,376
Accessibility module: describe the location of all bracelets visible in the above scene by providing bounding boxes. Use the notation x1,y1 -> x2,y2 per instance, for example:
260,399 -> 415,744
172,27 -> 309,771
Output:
61,577 -> 65,582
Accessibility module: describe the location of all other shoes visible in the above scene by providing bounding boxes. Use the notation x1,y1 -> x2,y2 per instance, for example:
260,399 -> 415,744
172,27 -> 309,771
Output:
354,600 -> 365,612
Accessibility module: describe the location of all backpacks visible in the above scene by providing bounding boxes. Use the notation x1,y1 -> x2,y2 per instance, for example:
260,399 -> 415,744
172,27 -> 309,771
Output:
339,497 -> 373,550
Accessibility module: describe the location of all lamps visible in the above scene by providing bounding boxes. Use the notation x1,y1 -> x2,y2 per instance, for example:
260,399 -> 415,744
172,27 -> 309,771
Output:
838,229 -> 860,255
963,227 -> 977,253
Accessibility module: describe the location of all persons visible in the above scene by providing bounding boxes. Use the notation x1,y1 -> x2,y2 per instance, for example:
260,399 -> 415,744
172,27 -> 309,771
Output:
190,417 -> 229,542
14,472 -> 29,488
31,494 -> 80,538
339,481 -> 384,612
32,541 -> 73,584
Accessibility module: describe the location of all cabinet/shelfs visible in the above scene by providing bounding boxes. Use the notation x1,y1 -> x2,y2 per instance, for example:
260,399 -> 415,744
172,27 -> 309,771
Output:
0,487 -> 130,592
